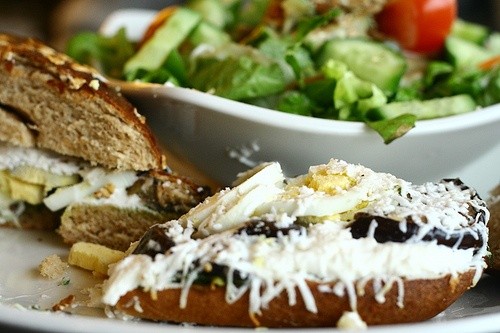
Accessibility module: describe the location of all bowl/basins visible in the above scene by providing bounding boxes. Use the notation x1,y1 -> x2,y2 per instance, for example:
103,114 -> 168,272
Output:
116,81 -> 500,186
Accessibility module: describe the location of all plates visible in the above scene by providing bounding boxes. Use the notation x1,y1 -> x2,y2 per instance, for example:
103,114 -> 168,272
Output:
1,225 -> 500,333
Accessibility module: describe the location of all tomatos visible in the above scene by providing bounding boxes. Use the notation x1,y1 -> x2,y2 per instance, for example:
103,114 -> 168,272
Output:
378,0 -> 455,54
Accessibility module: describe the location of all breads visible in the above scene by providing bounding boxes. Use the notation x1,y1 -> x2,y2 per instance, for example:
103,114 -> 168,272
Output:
0,31 -> 209,246
106,156 -> 489,328
